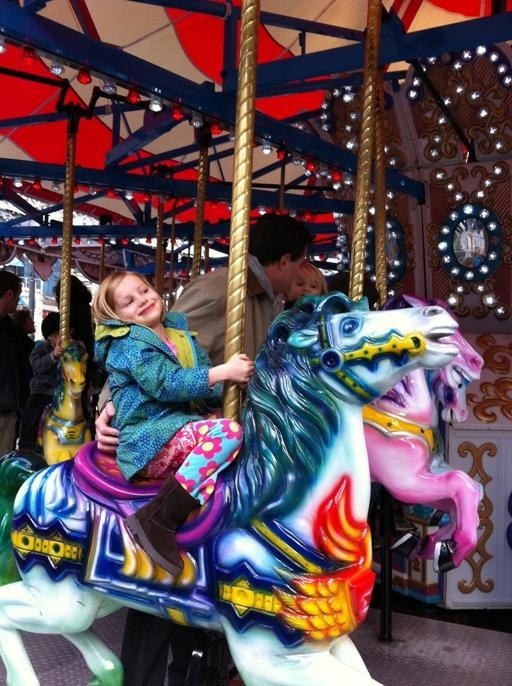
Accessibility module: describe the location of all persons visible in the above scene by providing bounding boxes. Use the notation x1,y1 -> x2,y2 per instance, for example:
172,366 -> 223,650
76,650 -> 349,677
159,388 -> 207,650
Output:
1,270 -> 92,458
287,260 -> 327,300
91,270 -> 254,569
92,215 -> 317,686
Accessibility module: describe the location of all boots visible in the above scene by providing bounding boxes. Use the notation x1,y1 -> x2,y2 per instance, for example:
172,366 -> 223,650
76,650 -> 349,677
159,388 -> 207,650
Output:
125,469 -> 201,576
